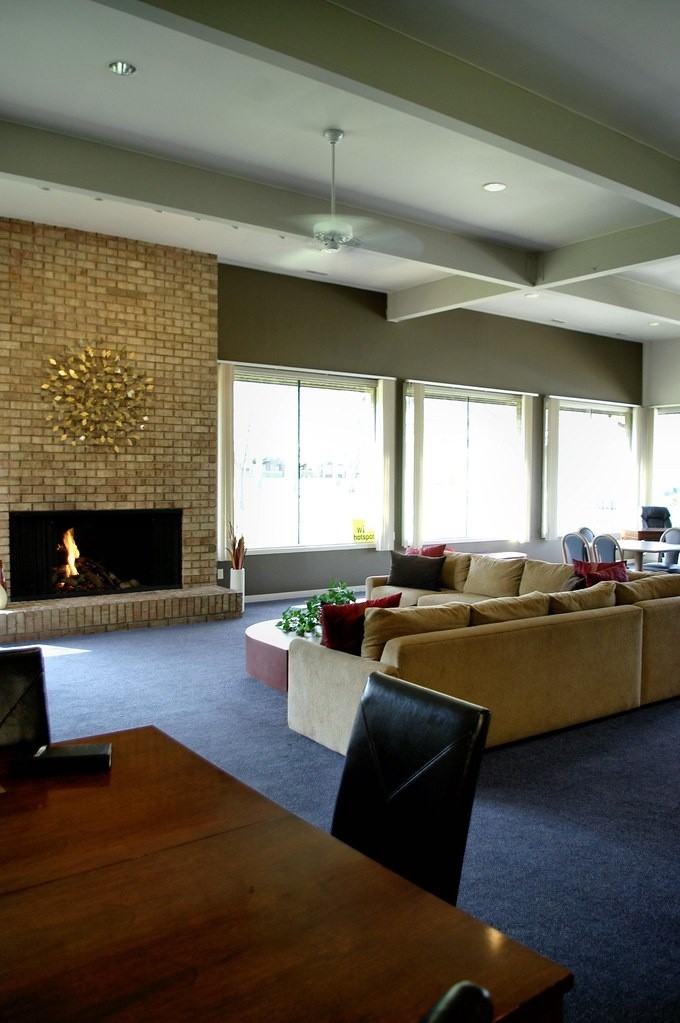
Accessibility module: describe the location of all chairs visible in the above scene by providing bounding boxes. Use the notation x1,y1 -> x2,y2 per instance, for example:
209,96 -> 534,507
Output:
330,671 -> 493,910
561,524 -> 680,574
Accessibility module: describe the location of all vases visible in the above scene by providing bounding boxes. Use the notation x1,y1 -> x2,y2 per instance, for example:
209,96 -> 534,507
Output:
226,568 -> 246,615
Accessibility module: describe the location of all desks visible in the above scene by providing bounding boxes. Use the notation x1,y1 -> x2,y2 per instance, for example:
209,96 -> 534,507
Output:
582,539 -> 680,571
0,724 -> 575,1023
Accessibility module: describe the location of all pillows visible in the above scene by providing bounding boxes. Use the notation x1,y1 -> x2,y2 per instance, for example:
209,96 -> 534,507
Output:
573,559 -> 627,578
586,562 -> 628,589
322,592 -> 403,657
547,580 -> 617,614
468,590 -> 550,626
385,550 -> 448,590
616,574 -> 680,604
560,572 -> 585,591
405,544 -> 446,557
441,552 -> 472,594
519,560 -> 575,596
463,555 -> 527,598
362,601 -> 470,661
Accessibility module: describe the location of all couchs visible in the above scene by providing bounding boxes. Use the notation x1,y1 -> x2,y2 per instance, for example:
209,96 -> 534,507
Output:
288,553 -> 680,758
